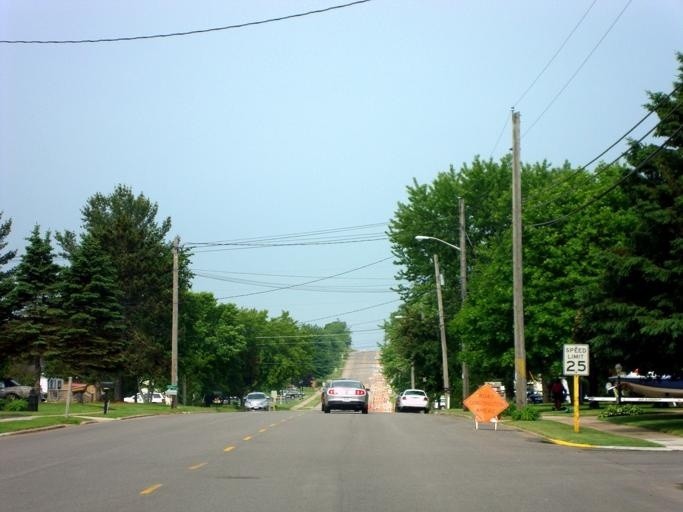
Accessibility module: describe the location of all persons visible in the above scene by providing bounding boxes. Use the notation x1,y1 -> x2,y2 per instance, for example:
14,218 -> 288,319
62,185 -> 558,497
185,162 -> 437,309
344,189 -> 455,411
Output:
550,377 -> 566,410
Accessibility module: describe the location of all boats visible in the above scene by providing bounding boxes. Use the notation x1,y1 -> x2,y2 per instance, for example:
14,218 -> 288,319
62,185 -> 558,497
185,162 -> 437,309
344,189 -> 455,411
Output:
584,370 -> 682,409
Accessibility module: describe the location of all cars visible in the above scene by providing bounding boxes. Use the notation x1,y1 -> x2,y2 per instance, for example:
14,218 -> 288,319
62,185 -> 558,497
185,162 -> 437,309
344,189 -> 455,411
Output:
525,387 -> 544,404
320,377 -> 370,413
39,385 -> 48,402
241,377 -> 302,411
394,388 -> 445,413
0,377 -> 36,401
123,392 -> 172,405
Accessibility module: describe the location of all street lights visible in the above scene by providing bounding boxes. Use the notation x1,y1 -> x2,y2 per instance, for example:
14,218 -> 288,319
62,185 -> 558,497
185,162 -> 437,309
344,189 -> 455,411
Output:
612,362 -> 623,407
390,314 -> 415,389
411,233 -> 472,415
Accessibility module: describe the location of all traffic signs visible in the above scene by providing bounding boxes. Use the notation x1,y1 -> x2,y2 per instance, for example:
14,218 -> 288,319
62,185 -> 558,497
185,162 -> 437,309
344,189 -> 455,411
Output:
560,342 -> 590,378
